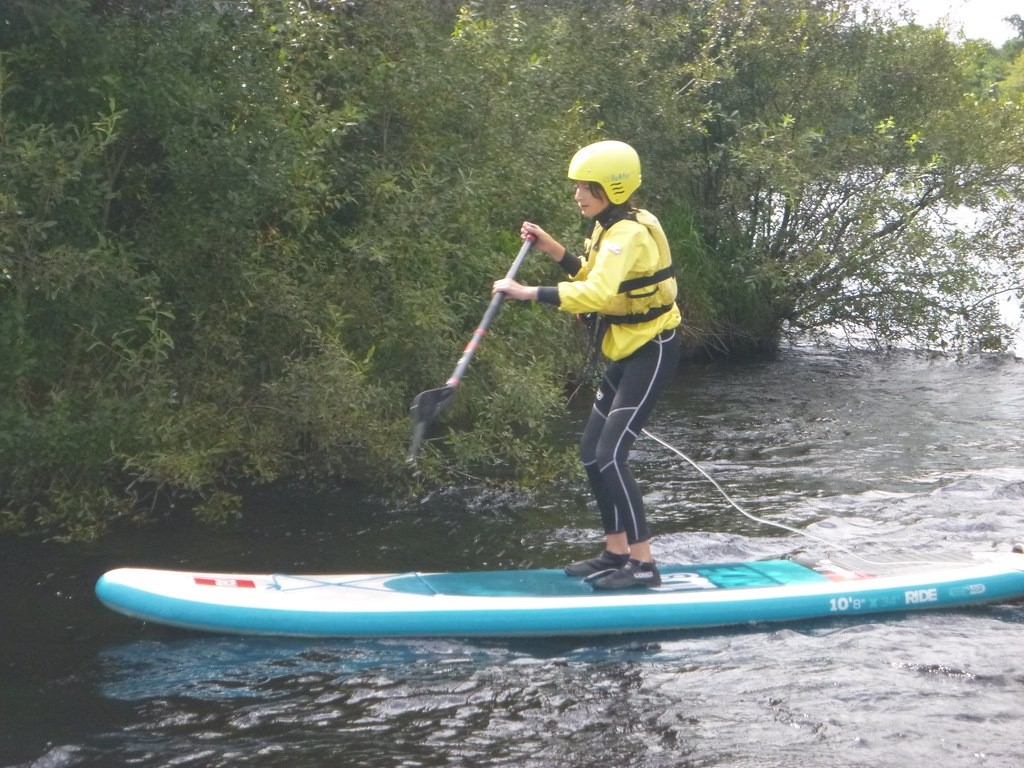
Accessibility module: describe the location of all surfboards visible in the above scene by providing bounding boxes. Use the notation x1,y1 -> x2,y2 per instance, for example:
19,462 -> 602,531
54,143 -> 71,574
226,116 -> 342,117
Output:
93,544 -> 1021,643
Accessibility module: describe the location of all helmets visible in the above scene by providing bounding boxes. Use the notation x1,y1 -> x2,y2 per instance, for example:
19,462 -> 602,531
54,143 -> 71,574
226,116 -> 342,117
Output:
567,140 -> 644,205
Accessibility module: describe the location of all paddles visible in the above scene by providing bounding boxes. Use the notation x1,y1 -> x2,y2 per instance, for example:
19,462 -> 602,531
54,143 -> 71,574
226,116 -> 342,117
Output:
409,225 -> 541,461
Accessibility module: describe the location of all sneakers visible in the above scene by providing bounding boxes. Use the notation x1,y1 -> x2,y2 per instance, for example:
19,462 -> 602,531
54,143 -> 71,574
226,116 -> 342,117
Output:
592,559 -> 662,589
565,550 -> 629,575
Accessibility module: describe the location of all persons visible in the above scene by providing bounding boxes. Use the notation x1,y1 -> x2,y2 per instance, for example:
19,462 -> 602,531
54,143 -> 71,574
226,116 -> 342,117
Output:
492,139 -> 685,591
